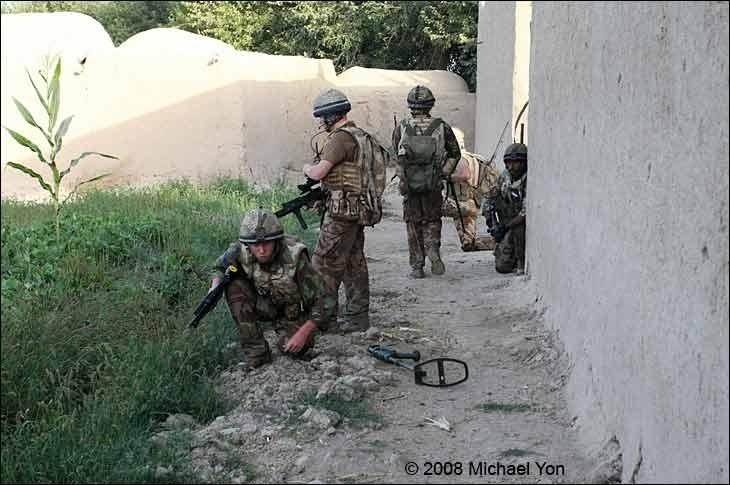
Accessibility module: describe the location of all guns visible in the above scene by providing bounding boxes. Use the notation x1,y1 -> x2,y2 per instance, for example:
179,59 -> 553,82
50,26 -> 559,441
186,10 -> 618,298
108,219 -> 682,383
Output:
366,343 -> 469,388
187,265 -> 237,329
271,177 -> 321,230
486,198 -> 505,242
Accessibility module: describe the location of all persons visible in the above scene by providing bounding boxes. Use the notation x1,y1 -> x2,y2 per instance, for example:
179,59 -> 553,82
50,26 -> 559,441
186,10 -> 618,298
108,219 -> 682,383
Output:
441,124 -> 493,253
389,83 -> 461,278
302,87 -> 373,333
209,207 -> 329,370
480,142 -> 527,274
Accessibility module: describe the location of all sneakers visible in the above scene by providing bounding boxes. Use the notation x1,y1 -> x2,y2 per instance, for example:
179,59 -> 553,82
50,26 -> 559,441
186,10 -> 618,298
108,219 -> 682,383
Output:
329,315 -> 369,332
241,351 -> 271,371
406,261 -> 445,279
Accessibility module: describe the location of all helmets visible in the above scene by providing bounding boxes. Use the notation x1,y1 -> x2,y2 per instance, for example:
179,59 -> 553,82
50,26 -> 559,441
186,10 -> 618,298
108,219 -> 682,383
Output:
406,84 -> 436,109
238,208 -> 285,244
503,143 -> 527,162
312,87 -> 351,117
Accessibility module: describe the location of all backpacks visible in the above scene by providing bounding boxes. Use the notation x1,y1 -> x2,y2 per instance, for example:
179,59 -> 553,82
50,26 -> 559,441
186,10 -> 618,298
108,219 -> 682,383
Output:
399,117 -> 446,195
